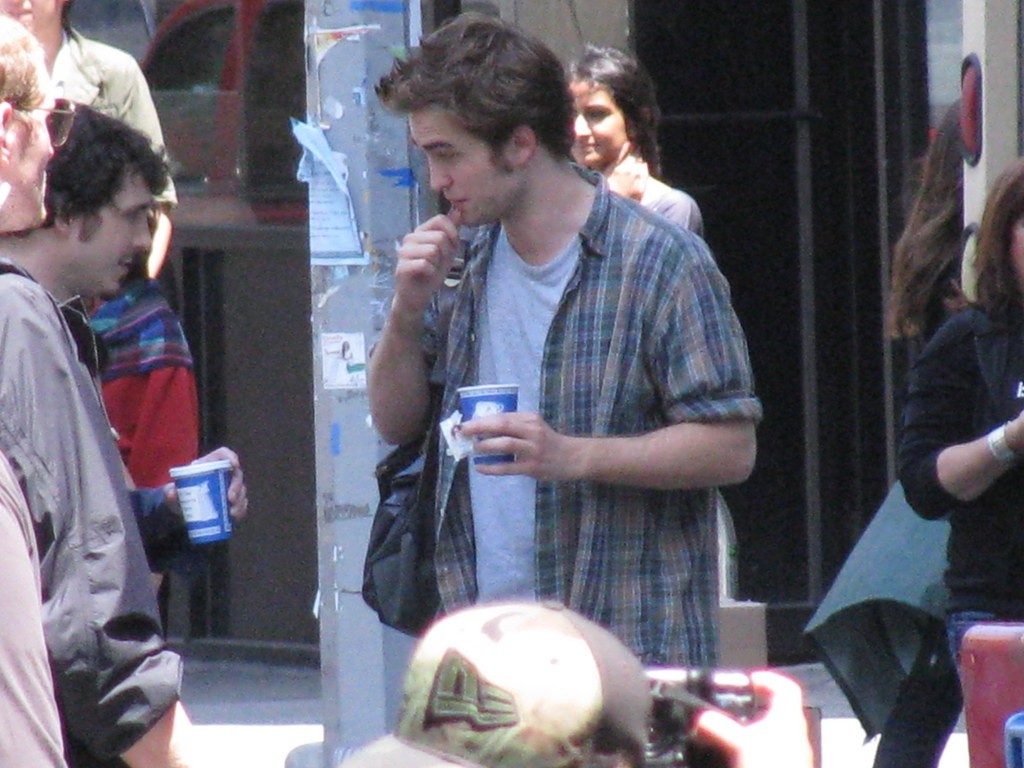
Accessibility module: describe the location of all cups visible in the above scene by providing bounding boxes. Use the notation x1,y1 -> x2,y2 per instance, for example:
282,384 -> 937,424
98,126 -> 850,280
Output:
456,385 -> 519,465
169,459 -> 233,545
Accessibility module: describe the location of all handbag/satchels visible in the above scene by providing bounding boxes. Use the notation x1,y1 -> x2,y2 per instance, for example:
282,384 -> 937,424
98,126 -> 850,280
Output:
362,236 -> 469,638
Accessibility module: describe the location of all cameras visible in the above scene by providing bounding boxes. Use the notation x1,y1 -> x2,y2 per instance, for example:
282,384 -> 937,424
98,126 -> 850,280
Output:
641,670 -> 755,768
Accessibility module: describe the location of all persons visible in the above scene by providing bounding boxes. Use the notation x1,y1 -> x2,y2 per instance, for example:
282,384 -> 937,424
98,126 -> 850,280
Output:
325,595 -> 820,768
363,12 -> 763,667
0,0 -> 179,279
80,254 -> 200,642
564,45 -> 746,602
0,451 -> 66,768
0,96 -> 247,768
805,99 -> 1024,768
0,8 -> 74,238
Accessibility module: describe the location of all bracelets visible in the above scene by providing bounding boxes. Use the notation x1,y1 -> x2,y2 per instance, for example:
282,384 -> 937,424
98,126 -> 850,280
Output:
990,422 -> 1013,466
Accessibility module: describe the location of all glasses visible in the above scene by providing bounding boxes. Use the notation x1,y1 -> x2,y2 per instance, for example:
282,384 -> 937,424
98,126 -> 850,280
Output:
4,98 -> 76,147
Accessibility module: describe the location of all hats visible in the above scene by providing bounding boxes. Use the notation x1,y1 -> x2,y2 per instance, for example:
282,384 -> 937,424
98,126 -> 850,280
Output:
342,599 -> 652,768
